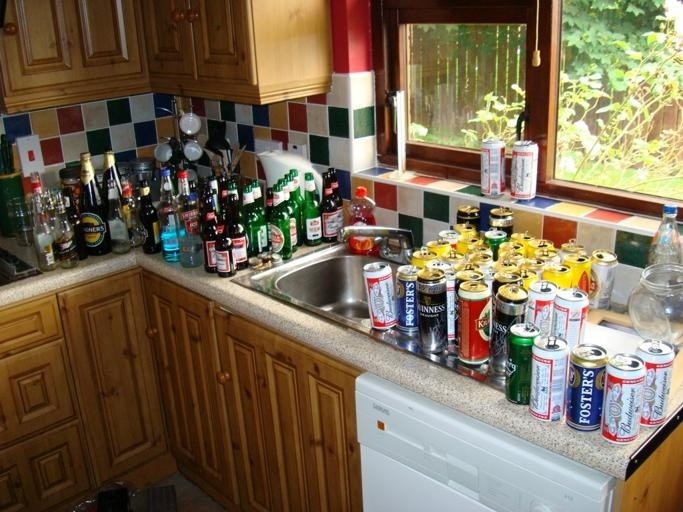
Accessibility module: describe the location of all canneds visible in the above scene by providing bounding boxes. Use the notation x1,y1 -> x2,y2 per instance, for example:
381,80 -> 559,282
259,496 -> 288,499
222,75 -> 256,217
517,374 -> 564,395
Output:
454,271 -> 485,348
363,262 -> 397,330
565,343 -> 609,432
509,140 -> 539,200
588,248 -> 619,310
600,352 -> 647,445
458,280 -> 492,365
635,338 -> 675,427
396,265 -> 427,332
59,156 -> 156,212
505,322 -> 541,405
480,140 -> 506,198
528,331 -> 569,423
550,288 -> 590,348
489,283 -> 530,380
416,268 -> 449,354
411,205 -> 591,317
526,280 -> 558,337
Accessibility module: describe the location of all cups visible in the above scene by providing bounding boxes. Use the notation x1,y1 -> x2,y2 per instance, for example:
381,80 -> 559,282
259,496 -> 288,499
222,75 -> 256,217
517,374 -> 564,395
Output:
6,197 -> 33,246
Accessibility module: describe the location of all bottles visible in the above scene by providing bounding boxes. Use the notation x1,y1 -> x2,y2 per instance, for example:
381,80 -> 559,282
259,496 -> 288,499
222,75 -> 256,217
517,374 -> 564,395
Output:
646,202 -> 682,268
347,185 -> 375,227
158,167 -> 344,279
27,149 -> 161,270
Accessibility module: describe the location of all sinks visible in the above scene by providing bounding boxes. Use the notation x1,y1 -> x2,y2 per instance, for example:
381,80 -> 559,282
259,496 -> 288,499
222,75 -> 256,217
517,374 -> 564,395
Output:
230,241 -> 408,320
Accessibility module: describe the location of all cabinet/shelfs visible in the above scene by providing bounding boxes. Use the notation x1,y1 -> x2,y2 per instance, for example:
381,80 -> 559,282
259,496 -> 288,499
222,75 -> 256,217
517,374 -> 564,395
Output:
212,306 -> 362,512
189,0 -> 333,105
142,1 -> 196,96
56,264 -> 170,500
0,1 -> 148,112
139,253 -> 240,512
0,288 -> 96,512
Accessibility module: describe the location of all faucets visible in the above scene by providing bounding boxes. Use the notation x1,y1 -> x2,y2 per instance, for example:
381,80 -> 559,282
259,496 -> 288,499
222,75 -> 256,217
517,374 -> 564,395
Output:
336,225 -> 417,265
